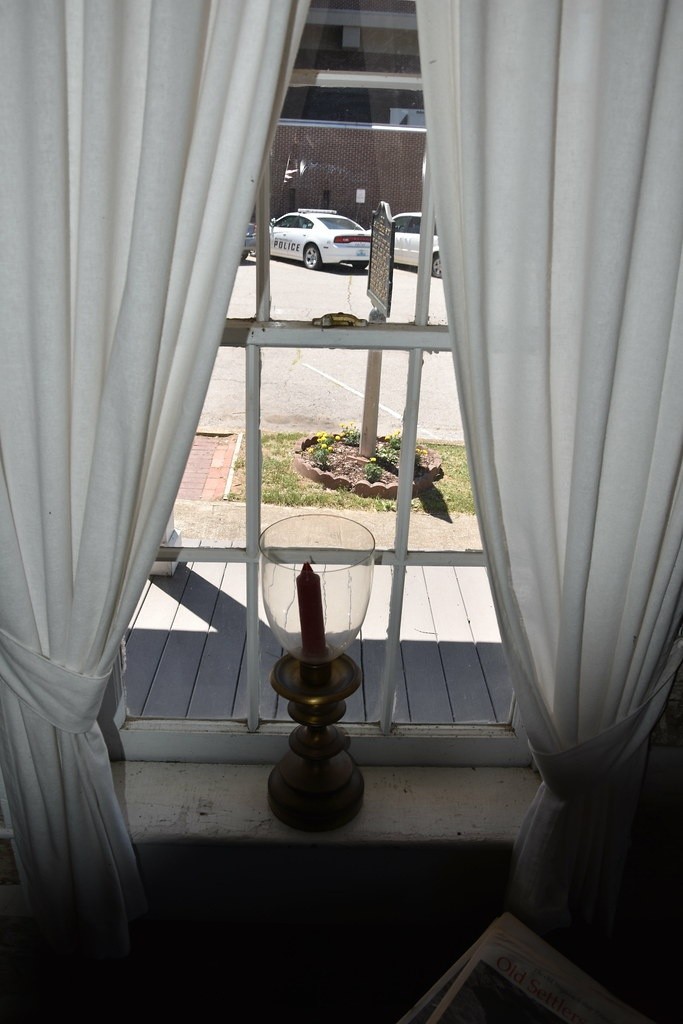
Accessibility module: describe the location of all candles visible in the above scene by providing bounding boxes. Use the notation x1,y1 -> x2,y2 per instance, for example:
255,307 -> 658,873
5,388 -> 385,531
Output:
298,561 -> 326,655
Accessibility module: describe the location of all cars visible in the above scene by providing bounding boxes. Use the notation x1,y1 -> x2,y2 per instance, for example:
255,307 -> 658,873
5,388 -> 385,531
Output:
368,211 -> 443,280
251,209 -> 372,270
239,223 -> 259,264
